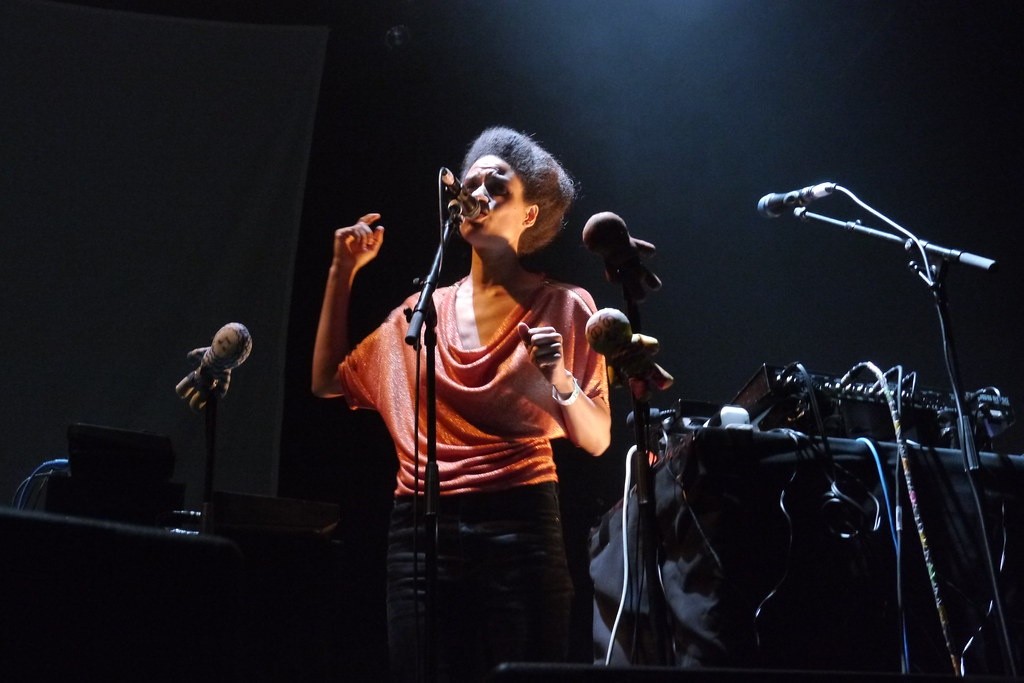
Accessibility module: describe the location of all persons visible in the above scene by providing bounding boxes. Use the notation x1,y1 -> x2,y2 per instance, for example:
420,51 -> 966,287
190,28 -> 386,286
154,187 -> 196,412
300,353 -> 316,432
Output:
311,127 -> 611,683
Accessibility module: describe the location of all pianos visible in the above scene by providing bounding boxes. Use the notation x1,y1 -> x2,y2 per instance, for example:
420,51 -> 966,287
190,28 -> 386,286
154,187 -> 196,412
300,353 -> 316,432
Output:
701,363 -> 1018,454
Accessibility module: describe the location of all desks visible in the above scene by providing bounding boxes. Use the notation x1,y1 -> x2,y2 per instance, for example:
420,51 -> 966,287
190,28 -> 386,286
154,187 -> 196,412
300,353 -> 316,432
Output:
588,425 -> 1023,676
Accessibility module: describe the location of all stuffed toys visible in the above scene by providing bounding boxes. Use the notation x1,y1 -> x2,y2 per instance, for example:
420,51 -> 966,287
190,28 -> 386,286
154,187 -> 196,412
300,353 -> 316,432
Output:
583,308 -> 674,399
582,211 -> 663,302
176,323 -> 252,412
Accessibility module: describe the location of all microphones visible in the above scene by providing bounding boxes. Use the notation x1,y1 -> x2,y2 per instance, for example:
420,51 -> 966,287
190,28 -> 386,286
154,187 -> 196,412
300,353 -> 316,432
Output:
757,183 -> 835,218
440,167 -> 482,219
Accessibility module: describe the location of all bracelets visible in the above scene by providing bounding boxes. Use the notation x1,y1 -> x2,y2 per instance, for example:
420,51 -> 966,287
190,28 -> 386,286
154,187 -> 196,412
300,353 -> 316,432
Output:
550,370 -> 579,407
